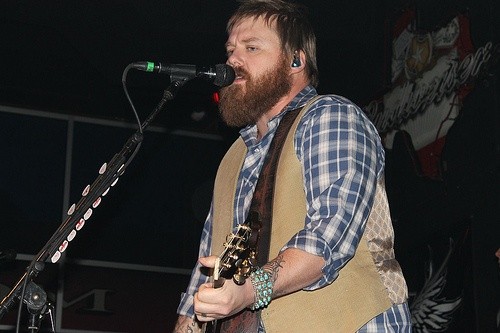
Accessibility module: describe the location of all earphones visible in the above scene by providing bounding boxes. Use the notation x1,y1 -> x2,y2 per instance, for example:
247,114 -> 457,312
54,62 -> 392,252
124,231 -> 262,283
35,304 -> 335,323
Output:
292,56 -> 301,67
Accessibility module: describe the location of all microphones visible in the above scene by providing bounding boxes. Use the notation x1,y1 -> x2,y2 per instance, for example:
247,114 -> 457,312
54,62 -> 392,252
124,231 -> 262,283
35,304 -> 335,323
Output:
133,60 -> 236,87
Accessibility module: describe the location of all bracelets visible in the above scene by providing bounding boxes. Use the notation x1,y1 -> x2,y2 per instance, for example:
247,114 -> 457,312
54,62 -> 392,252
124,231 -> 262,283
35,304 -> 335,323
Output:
249,267 -> 274,311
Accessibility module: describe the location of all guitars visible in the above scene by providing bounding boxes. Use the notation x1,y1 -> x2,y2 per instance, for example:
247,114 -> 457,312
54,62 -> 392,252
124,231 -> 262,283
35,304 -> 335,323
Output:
201,224 -> 253,333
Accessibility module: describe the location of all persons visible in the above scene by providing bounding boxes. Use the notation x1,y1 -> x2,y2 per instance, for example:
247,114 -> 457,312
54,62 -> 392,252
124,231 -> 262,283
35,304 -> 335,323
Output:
173,0 -> 413,333
